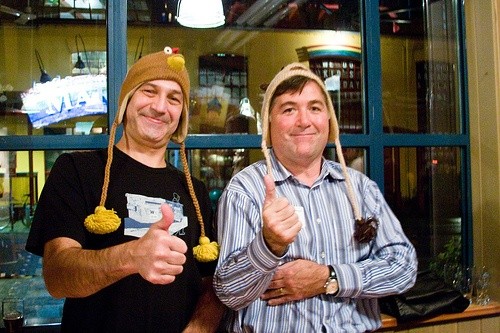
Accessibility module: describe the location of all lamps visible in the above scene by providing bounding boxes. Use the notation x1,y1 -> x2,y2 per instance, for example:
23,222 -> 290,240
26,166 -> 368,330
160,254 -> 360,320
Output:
33,49 -> 52,83
175,0 -> 226,28
134,36 -> 145,64
74,34 -> 92,74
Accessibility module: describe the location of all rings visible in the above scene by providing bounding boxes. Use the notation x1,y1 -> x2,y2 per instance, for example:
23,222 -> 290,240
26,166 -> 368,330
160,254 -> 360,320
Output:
279,288 -> 283,294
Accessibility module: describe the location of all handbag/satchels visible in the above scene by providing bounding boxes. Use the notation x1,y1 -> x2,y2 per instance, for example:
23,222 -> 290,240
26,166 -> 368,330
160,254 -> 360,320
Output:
380,269 -> 469,329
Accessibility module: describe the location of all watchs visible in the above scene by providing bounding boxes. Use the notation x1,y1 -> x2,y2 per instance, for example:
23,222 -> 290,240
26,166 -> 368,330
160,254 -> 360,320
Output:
326,265 -> 340,295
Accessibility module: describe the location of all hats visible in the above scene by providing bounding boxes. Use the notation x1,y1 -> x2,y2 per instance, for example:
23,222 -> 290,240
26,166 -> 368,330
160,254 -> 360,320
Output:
260,60 -> 379,243
84,46 -> 219,260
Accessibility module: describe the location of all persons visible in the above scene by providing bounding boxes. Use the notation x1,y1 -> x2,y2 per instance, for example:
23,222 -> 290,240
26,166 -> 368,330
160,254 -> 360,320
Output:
26,47 -> 226,333
213,62 -> 417,333
